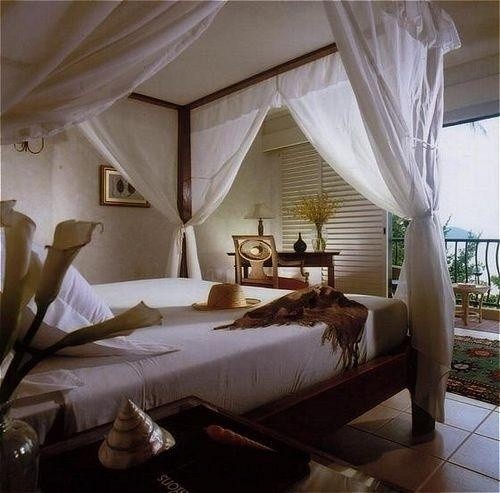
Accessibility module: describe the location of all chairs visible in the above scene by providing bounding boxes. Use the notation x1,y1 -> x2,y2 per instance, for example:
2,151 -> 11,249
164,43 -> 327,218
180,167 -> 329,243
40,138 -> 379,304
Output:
231,232 -> 280,289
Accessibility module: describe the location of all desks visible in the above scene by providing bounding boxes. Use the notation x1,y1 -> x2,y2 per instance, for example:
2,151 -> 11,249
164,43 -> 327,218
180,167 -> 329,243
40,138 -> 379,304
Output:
451,282 -> 490,325
226,249 -> 341,291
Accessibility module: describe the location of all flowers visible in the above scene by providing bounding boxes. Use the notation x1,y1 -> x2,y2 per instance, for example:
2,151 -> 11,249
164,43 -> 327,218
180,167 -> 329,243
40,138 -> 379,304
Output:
280,188 -> 349,251
0,195 -> 164,409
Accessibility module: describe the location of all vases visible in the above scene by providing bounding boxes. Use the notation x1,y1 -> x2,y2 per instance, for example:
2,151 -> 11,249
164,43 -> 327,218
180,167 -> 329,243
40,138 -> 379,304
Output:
291,230 -> 309,251
2,406 -> 46,493
311,220 -> 328,250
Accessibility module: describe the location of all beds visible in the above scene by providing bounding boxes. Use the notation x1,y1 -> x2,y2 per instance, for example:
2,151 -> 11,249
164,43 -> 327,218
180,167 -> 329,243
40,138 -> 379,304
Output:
0,270 -> 443,452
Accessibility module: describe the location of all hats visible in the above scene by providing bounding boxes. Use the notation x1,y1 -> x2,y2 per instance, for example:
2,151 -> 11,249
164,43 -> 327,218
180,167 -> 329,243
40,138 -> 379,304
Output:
193,283 -> 261,311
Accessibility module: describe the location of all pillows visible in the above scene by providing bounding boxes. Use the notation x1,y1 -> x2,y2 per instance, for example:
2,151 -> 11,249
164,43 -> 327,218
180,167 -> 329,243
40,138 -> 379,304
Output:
0,223 -> 184,358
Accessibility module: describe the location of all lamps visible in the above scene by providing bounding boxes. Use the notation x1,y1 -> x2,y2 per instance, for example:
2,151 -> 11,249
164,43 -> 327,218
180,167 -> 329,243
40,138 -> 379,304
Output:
245,202 -> 275,234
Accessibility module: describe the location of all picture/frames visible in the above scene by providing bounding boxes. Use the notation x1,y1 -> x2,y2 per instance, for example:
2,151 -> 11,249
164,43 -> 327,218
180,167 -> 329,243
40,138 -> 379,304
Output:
96,161 -> 153,208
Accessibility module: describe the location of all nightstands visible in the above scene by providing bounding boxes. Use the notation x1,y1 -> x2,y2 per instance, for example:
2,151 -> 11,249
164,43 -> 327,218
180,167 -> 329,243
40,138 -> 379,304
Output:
31,391 -> 395,493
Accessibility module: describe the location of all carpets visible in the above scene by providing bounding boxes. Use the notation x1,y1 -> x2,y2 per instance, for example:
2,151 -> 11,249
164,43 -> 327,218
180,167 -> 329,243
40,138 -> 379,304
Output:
445,332 -> 499,408
